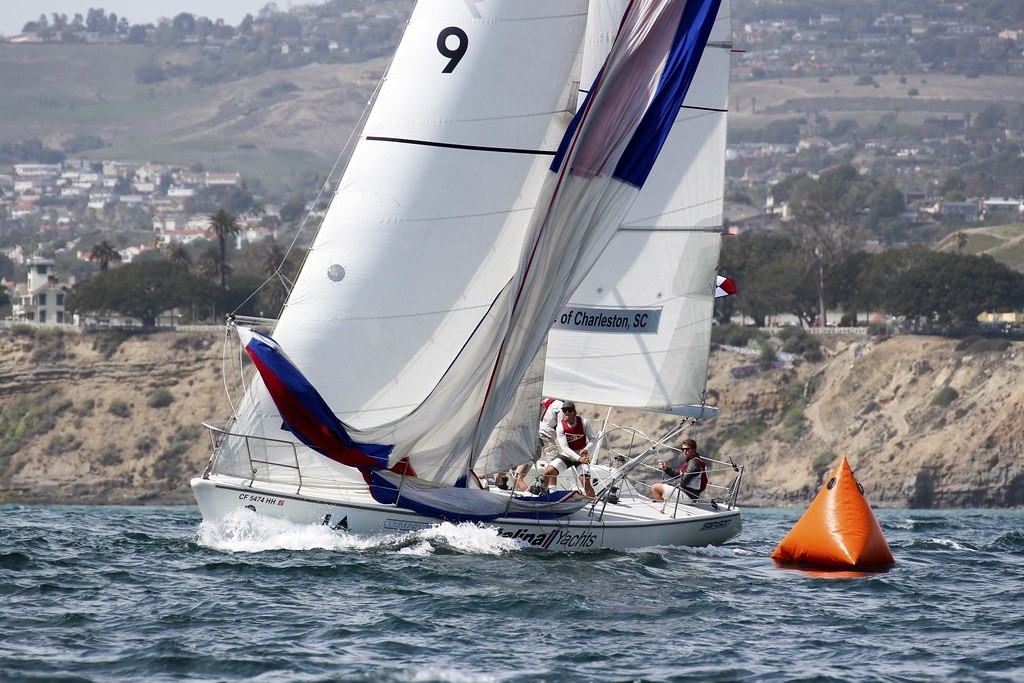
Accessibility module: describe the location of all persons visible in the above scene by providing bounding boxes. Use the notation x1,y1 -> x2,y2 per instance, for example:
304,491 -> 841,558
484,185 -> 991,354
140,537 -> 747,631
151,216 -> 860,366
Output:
495,398 -> 597,498
651,439 -> 708,503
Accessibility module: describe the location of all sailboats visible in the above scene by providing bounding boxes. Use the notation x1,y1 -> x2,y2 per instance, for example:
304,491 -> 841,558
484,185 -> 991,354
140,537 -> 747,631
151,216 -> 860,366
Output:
192,0 -> 744,553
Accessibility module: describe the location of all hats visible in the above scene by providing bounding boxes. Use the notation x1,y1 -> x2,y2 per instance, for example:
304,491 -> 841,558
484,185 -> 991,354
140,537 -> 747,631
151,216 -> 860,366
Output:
561,401 -> 574,408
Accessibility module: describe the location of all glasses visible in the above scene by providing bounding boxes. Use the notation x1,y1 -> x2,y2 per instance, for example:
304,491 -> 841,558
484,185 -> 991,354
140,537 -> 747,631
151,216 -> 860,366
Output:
562,408 -> 573,412
681,448 -> 693,451
